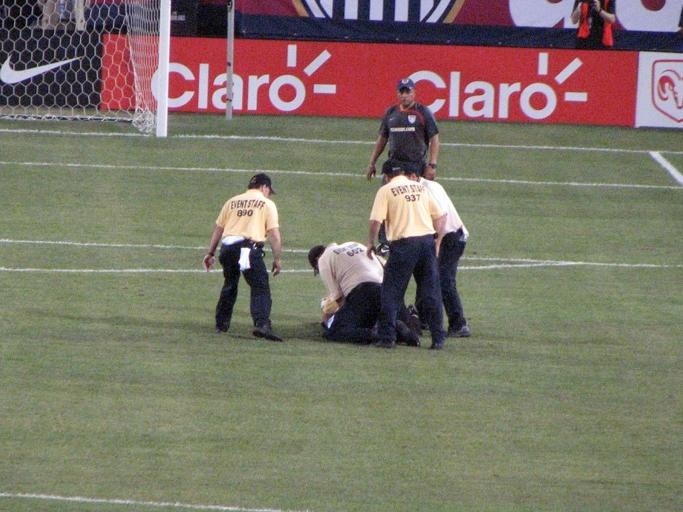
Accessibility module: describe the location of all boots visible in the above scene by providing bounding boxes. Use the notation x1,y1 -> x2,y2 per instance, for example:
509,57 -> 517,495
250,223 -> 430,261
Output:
252,318 -> 282,341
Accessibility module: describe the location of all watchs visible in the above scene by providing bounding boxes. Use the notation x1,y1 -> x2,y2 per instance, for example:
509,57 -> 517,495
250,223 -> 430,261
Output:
429,163 -> 437,168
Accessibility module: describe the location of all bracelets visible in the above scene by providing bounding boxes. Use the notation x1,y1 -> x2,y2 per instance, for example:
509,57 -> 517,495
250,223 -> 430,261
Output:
208,252 -> 214,256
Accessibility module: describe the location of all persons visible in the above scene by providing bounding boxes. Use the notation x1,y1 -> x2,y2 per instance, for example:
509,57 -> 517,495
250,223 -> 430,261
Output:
678,6 -> 683,33
308,160 -> 471,351
570,0 -> 616,50
202,175 -> 282,340
366,78 -> 440,256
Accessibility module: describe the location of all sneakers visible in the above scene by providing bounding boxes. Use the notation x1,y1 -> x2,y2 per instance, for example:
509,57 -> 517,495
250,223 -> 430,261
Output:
377,244 -> 388,255
375,304 -> 470,349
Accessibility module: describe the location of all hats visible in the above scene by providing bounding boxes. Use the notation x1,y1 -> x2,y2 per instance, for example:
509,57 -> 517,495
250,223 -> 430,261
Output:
397,78 -> 414,89
249,173 -> 275,194
308,246 -> 322,276
381,159 -> 416,175
